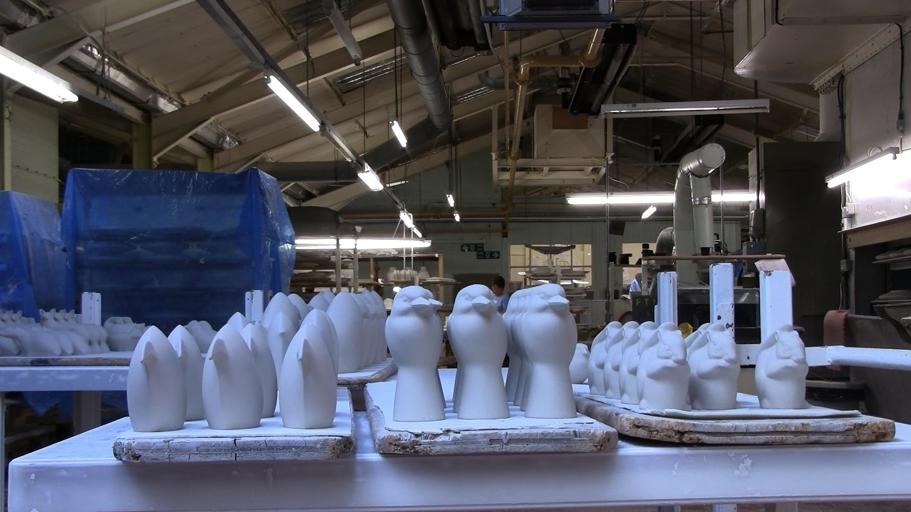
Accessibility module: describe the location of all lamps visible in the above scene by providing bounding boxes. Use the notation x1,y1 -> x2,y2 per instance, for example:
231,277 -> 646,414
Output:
261,68 -> 320,132
293,235 -> 432,251
445,192 -> 461,223
390,120 -> 407,148
355,162 -> 383,191
399,207 -> 423,239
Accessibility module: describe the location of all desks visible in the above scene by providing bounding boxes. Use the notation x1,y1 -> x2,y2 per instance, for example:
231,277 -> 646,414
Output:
3,361 -> 910,511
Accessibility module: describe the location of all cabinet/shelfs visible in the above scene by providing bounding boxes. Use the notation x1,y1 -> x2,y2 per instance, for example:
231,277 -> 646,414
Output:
292,253 -> 445,320
848,218 -> 911,323
527,244 -> 575,302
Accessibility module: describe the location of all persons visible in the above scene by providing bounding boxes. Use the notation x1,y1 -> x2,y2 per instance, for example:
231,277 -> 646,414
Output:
490,276 -> 510,314
631,273 -> 643,293
